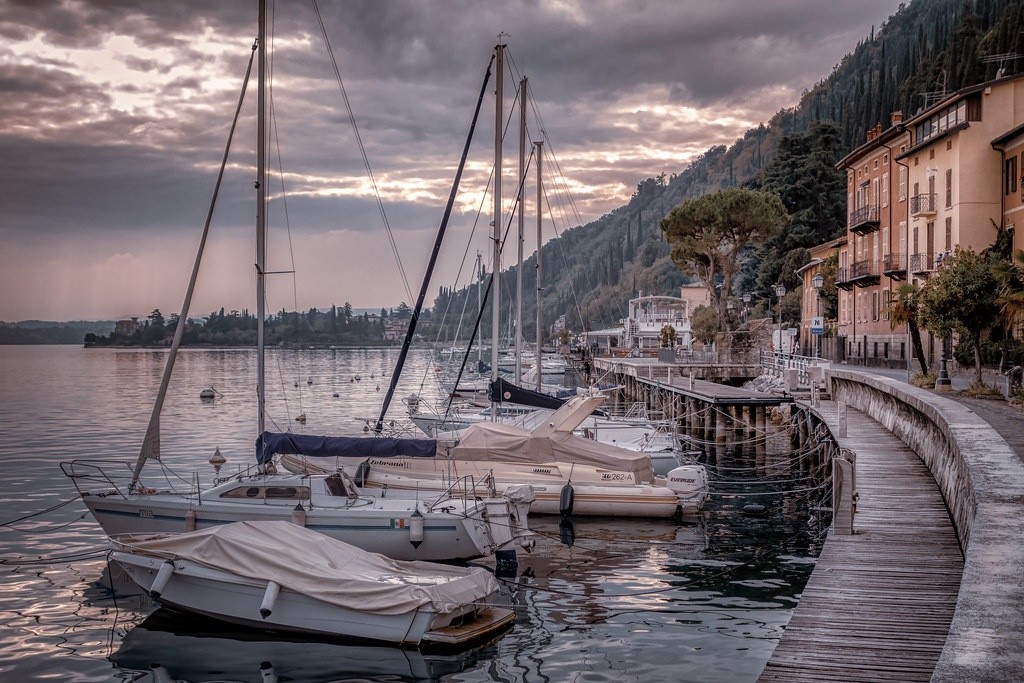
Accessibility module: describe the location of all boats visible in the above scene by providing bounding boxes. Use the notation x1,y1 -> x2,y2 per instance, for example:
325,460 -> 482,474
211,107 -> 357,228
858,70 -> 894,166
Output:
103,517 -> 519,650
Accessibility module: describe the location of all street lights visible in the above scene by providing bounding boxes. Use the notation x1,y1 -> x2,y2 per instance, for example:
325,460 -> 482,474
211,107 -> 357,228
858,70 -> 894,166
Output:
743,292 -> 752,322
776,285 -> 785,360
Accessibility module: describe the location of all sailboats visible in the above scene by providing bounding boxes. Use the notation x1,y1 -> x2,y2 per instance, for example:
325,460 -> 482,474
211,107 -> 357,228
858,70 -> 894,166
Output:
275,28 -> 711,526
63,0 -> 537,561
